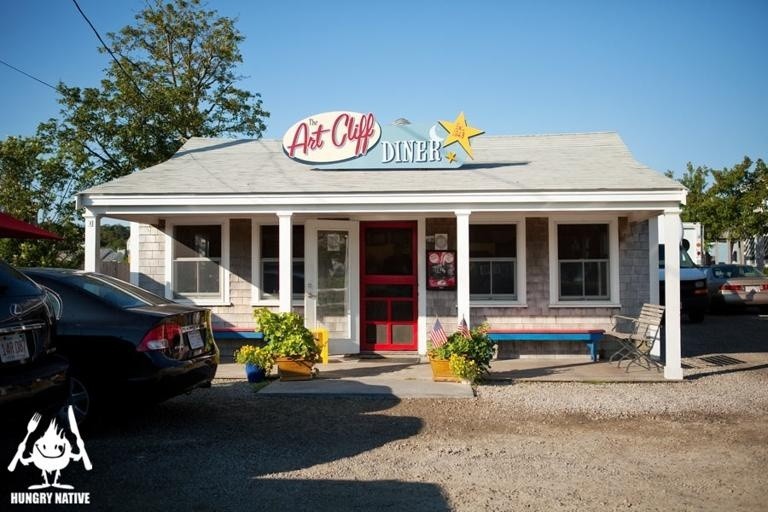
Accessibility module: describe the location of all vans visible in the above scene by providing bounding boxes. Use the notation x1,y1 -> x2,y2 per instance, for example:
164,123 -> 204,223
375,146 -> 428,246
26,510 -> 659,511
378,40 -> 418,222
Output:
660,243 -> 710,313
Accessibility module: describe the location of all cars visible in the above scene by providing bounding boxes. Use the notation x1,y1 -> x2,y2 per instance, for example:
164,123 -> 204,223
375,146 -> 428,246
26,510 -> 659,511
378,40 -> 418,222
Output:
707,265 -> 768,311
22,264 -> 220,427
0,258 -> 67,433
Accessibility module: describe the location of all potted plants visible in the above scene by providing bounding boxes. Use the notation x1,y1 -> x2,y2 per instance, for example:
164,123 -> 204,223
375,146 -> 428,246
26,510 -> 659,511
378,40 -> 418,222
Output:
234,346 -> 265,382
427,323 -> 495,385
255,307 -> 327,381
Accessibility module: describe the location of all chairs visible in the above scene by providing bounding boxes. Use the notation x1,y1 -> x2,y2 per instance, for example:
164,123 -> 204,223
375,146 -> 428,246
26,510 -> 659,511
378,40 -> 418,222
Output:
604,302 -> 665,374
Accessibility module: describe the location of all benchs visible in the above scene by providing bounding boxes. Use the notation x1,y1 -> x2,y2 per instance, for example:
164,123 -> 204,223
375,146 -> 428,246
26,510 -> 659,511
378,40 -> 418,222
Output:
486,329 -> 605,363
213,328 -> 264,346
